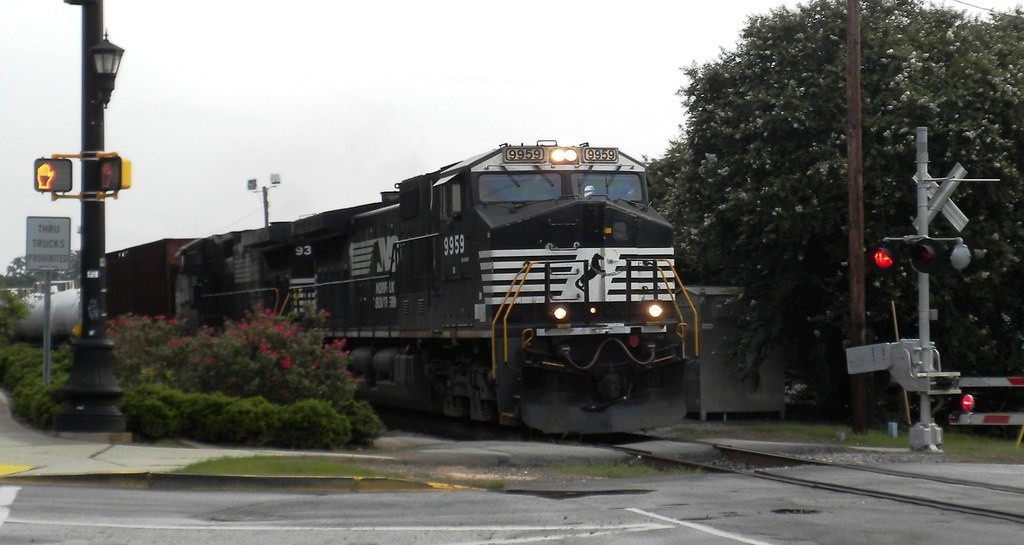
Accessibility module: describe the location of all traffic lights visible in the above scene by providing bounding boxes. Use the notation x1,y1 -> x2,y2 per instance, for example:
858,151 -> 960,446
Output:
909,238 -> 945,274
873,245 -> 896,274
95,154 -> 133,189
33,156 -> 73,192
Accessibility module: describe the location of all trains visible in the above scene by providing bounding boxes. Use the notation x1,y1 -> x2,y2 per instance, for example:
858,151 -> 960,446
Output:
1,141 -> 705,440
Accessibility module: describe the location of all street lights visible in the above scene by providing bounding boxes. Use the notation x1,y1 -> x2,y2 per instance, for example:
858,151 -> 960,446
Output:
247,173 -> 281,228
53,29 -> 125,431
